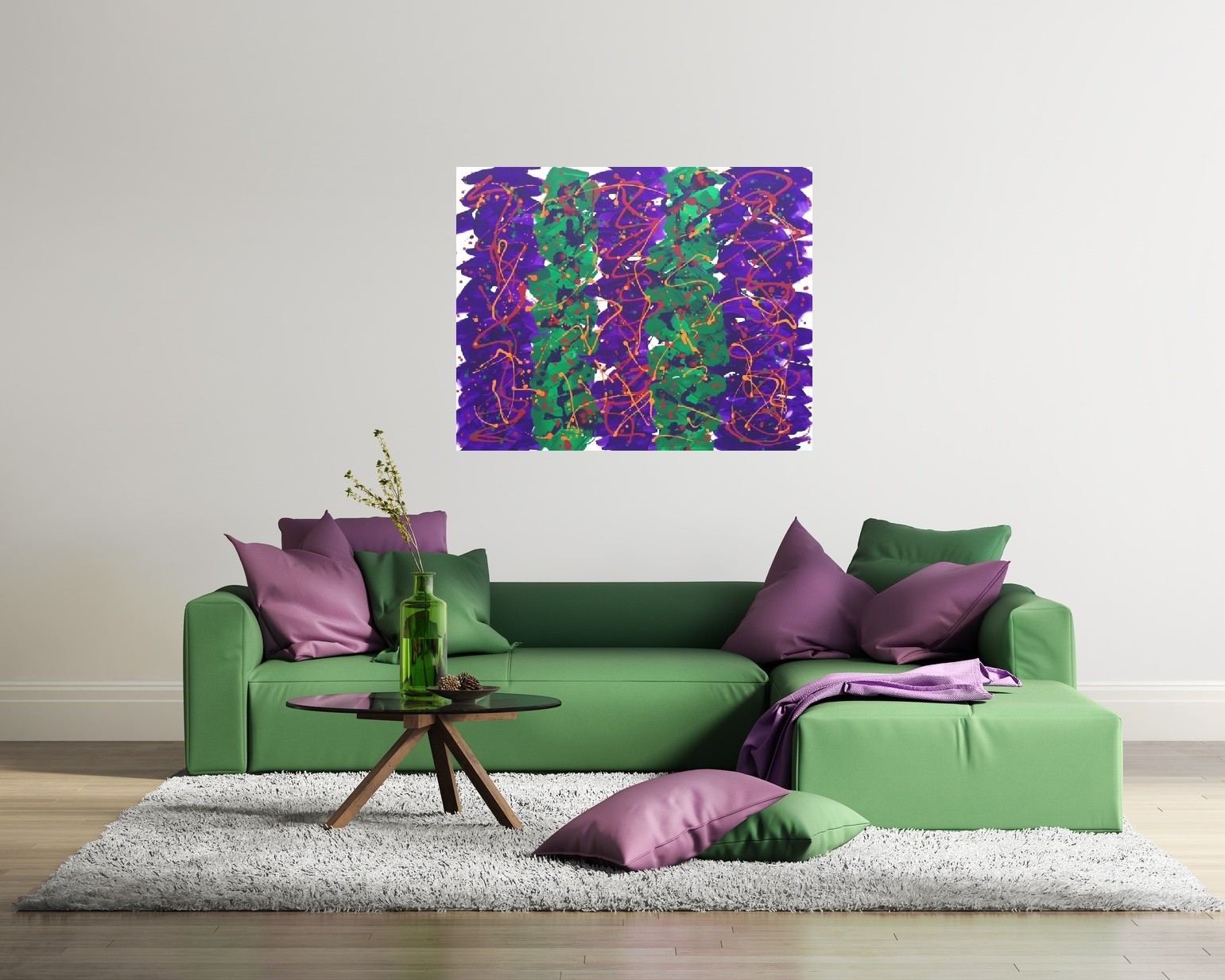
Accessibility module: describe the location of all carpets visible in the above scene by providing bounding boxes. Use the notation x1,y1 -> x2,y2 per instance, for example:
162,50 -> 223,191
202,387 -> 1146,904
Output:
25,775 -> 1224,918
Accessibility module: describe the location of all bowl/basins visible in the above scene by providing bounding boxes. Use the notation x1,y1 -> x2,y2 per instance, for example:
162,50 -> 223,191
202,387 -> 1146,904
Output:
426,686 -> 502,704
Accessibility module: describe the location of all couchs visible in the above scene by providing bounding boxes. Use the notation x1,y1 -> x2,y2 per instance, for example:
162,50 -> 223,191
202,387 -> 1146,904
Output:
182,583 -> 1123,833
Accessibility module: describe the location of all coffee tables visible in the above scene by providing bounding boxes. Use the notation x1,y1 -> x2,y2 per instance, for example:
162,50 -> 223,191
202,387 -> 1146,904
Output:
285,692 -> 563,829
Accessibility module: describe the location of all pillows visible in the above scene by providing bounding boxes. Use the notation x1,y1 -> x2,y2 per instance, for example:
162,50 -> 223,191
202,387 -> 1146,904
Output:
532,769 -> 791,872
225,508 -> 522,662
722,515 -> 1012,659
695,790 -> 872,860
